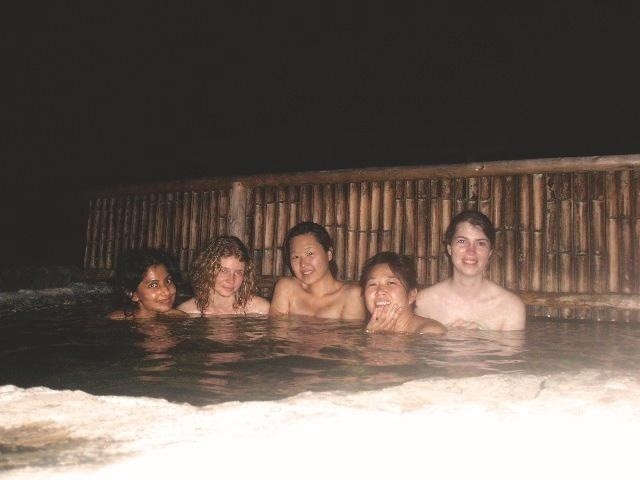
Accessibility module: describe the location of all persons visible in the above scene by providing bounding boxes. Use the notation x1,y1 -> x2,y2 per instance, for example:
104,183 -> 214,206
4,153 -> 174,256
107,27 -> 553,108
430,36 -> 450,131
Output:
414,211 -> 526,331
362,252 -> 449,333
106,246 -> 188,319
177,236 -> 271,314
270,223 -> 367,320
